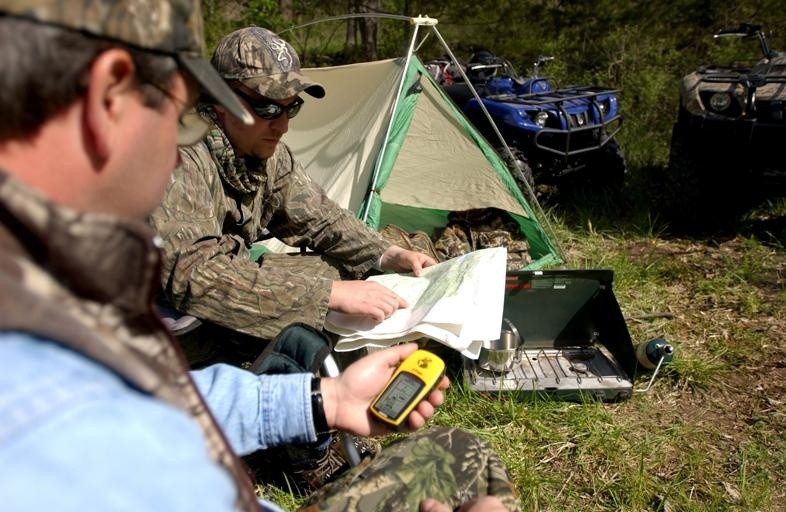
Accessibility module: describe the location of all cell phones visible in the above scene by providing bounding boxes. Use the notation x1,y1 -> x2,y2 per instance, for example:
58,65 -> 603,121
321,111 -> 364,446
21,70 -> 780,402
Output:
369,349 -> 447,431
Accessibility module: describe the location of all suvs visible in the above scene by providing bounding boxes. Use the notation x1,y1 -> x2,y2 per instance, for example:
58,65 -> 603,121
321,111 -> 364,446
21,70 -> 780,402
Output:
669,23 -> 785,208
423,43 -> 627,207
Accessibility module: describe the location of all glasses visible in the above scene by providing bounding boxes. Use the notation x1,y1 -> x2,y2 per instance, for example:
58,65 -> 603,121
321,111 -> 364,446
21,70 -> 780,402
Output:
234,86 -> 305,120
142,75 -> 216,148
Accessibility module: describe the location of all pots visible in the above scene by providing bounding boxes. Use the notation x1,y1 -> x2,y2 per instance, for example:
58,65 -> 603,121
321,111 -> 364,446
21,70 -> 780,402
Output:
478,317 -> 525,373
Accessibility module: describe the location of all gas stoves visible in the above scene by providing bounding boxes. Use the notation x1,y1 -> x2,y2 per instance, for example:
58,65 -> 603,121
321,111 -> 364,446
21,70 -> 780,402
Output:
462,347 -> 633,403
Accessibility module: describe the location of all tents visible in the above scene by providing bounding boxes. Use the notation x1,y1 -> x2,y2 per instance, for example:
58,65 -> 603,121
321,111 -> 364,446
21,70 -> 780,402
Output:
250,13 -> 569,270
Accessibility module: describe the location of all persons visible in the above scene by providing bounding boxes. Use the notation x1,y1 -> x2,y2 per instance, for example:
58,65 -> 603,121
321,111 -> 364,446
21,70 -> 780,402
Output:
152,26 -> 437,489
0,0 -> 521,512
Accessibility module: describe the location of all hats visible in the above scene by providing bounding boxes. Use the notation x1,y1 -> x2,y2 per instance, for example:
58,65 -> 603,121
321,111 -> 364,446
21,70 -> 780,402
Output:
211,27 -> 325,100
0,1 -> 255,127
